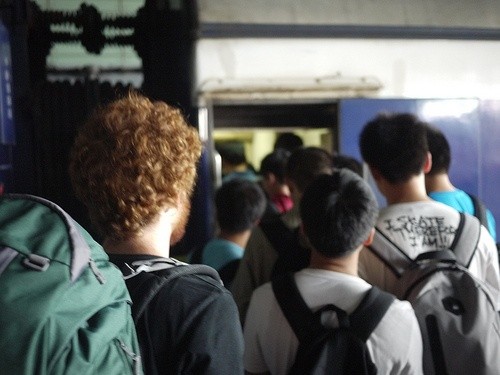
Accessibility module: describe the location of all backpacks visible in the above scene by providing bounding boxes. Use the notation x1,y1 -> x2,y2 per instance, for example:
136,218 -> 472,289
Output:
365,212 -> 500,375
271,271 -> 396,375
0,193 -> 224,375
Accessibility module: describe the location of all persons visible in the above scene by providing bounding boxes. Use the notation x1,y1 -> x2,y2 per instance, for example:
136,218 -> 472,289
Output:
259,150 -> 295,213
358,112 -> 500,312
214,139 -> 258,177
244,167 -> 423,375
333,156 -> 363,178
229,148 -> 333,310
423,122 -> 496,243
67,86 -> 245,375
186,177 -> 266,289
274,133 -> 303,151
